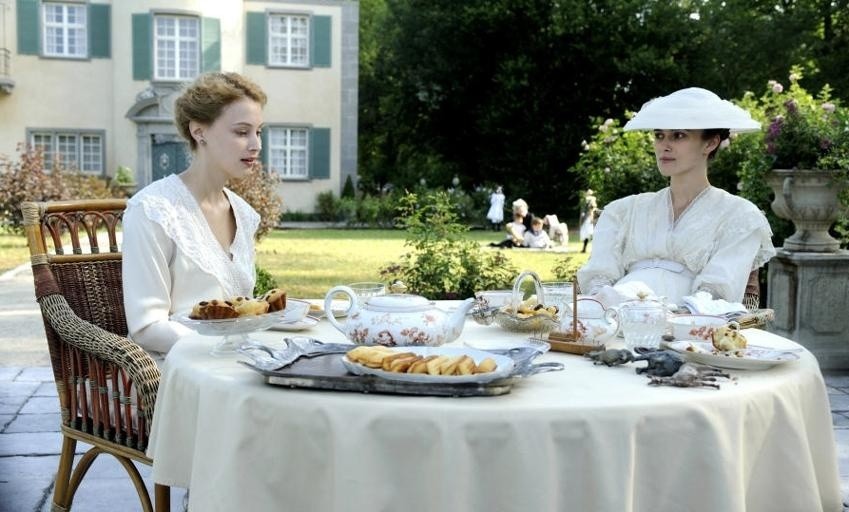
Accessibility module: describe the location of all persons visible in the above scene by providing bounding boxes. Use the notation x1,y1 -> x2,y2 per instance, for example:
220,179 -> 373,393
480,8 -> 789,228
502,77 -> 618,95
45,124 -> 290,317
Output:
505,213 -> 526,247
578,188 -> 596,253
485,185 -> 506,232
523,218 -> 551,249
121,71 -> 266,375
575,87 -> 776,315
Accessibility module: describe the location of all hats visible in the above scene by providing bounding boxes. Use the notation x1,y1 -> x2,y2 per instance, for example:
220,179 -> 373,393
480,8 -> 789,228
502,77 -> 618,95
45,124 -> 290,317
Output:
617,87 -> 763,133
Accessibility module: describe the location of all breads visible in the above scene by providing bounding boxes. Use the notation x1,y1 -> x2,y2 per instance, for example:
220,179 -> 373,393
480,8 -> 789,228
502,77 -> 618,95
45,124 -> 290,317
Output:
346,345 -> 497,377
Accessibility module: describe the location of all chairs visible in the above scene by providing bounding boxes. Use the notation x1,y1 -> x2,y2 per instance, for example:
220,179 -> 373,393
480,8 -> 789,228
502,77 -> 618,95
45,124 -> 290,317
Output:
732,268 -> 775,330
21,197 -> 169,512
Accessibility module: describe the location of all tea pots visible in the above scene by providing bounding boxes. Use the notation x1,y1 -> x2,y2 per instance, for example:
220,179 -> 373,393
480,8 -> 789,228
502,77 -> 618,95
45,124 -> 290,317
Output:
324,279 -> 475,346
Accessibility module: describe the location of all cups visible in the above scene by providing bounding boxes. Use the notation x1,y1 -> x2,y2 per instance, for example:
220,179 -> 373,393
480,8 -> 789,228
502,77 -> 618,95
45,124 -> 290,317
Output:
349,283 -> 385,310
619,306 -> 667,356
540,281 -> 573,319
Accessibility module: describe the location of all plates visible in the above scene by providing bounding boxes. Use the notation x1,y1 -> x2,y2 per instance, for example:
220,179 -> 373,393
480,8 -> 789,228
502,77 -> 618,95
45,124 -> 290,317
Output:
342,345 -> 515,385
431,298 -> 481,315
269,315 -> 321,332
299,298 -> 351,318
664,340 -> 799,369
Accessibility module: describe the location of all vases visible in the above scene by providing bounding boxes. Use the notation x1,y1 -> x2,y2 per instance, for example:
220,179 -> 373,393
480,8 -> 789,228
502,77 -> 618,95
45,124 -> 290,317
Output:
770,169 -> 845,251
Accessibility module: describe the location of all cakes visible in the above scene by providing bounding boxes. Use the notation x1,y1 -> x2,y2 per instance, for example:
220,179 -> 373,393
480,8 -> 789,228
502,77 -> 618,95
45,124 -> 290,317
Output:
199,306 -> 240,321
711,326 -> 748,354
236,299 -> 269,317
263,288 -> 287,313
189,299 -> 235,320
231,296 -> 252,305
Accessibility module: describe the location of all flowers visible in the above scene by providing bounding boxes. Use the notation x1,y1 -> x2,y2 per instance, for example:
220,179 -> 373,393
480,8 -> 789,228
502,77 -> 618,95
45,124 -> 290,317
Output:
722,61 -> 849,246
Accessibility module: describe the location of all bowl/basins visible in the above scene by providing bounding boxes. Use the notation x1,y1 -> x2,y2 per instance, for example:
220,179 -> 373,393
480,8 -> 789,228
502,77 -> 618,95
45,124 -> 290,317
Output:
475,290 -> 525,308
281,298 -> 311,323
667,313 -> 729,339
